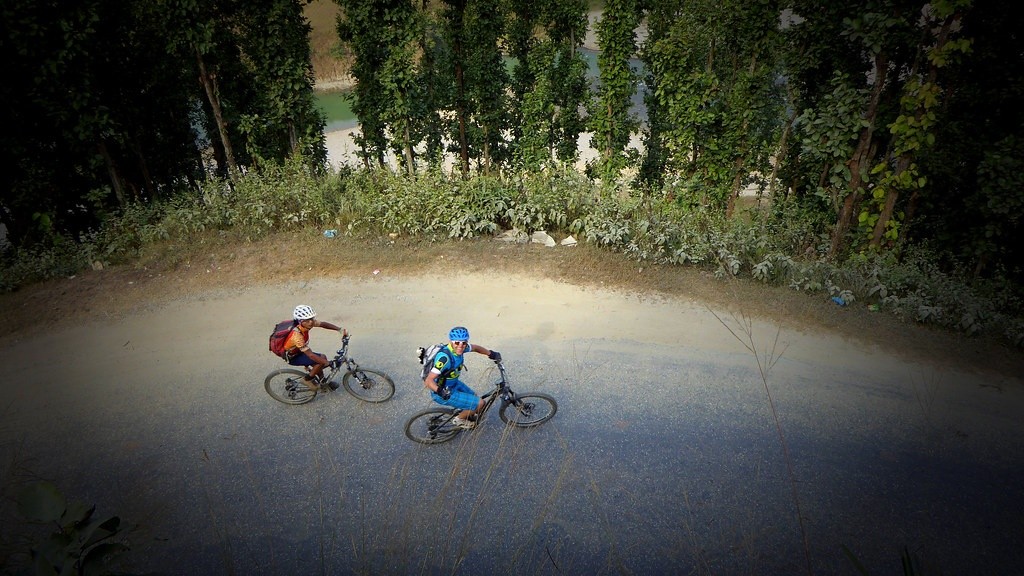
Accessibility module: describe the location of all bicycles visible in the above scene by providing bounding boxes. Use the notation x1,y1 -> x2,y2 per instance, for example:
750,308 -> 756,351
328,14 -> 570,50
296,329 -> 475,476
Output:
263,333 -> 396,406
404,354 -> 558,449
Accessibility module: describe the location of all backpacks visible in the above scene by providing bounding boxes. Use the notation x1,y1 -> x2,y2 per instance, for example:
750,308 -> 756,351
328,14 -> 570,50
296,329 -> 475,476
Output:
268,319 -> 304,364
416,342 -> 468,388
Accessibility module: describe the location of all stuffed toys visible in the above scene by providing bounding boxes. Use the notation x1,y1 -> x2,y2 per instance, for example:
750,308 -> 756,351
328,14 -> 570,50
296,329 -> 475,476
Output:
416,347 -> 425,364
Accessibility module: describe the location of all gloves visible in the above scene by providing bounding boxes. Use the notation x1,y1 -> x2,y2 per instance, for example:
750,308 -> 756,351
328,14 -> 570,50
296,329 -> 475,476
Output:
340,327 -> 352,340
488,349 -> 502,364
329,359 -> 342,369
437,386 -> 451,400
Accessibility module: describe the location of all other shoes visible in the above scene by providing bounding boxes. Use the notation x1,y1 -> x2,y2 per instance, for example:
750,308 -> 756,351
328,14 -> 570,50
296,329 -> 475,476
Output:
319,377 -> 339,390
300,376 -> 321,390
453,416 -> 475,428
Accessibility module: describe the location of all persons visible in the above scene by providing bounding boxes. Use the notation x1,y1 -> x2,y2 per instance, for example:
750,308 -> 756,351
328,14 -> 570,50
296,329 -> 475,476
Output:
420,327 -> 501,429
284,305 -> 349,391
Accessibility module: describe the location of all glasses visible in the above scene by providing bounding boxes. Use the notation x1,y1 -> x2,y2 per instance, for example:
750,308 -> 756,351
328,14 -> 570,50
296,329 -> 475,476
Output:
301,317 -> 314,323
452,341 -> 467,345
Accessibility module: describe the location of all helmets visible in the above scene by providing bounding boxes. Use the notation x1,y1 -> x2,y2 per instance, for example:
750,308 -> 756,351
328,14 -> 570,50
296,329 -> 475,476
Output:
293,305 -> 316,320
449,326 -> 469,341
